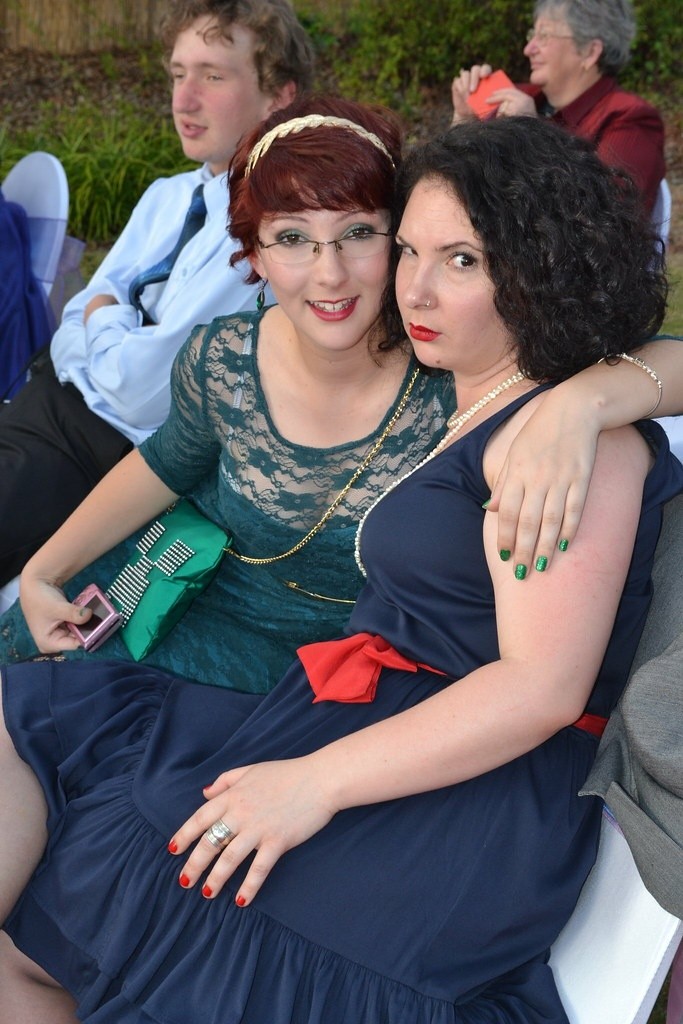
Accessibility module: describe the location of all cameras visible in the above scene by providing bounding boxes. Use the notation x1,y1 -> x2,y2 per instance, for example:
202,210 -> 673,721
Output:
67,583 -> 124,653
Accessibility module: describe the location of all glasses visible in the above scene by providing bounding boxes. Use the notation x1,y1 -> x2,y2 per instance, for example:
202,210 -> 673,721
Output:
257,225 -> 394,265
527,29 -> 579,47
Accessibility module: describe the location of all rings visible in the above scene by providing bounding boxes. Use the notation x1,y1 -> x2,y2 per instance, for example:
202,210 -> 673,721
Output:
207,819 -> 237,851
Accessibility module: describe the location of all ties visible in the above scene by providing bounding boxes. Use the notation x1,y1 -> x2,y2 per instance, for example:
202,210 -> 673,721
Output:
128,184 -> 206,327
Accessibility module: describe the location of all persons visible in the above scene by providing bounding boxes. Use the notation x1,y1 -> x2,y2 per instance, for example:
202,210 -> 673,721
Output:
0,1 -> 314,589
453,0 -> 666,222
1,90 -> 683,692
0,117 -> 683,1024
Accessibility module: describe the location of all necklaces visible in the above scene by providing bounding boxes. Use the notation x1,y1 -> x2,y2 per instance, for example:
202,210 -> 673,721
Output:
354,369 -> 533,577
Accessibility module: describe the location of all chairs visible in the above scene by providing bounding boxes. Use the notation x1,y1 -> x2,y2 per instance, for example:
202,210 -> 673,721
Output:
0,151 -> 70,298
547,487 -> 683,1024
648,177 -> 673,273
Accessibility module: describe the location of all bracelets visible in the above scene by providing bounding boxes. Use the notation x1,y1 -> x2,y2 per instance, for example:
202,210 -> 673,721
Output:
599,352 -> 664,418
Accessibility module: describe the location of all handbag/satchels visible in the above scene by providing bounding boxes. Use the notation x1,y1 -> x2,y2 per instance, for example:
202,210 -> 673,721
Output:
106,498 -> 235,663
